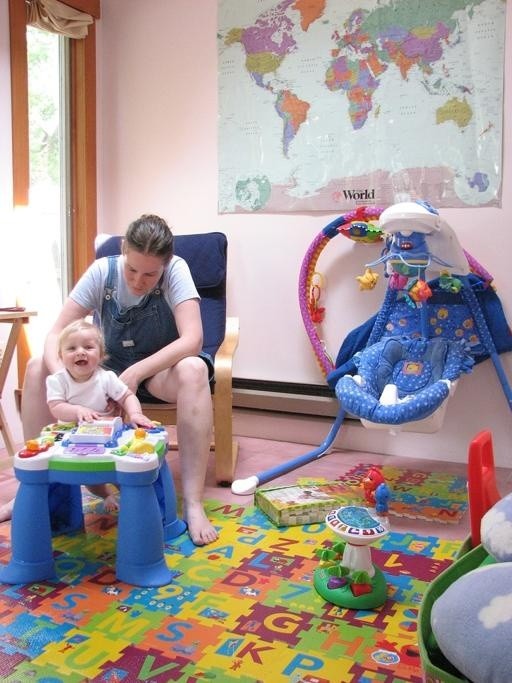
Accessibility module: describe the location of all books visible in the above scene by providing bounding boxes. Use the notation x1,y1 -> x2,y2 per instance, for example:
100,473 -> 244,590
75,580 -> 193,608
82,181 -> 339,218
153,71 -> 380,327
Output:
69,415 -> 123,444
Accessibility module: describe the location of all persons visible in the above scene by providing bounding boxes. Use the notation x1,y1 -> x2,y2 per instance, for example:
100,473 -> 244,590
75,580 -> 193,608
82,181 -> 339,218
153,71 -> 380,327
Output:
1,211 -> 222,549
44,318 -> 158,514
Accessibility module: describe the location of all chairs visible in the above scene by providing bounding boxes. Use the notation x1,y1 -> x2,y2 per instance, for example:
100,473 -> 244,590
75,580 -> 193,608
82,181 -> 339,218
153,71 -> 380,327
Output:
92,231 -> 241,486
